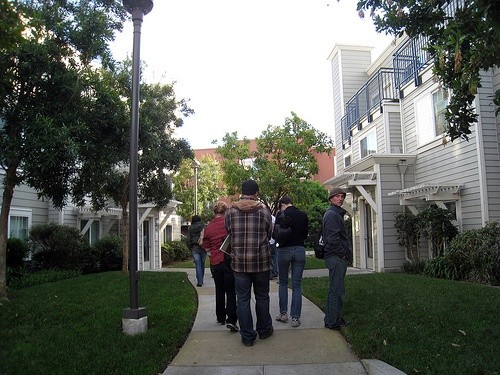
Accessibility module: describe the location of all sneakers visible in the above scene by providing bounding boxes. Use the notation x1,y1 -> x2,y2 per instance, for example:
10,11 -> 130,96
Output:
275,313 -> 288,321
225,323 -> 240,332
291,317 -> 301,327
216,320 -> 225,325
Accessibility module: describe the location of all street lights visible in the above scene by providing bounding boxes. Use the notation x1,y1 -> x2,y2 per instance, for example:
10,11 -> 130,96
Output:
121,0 -> 154,335
190,160 -> 202,215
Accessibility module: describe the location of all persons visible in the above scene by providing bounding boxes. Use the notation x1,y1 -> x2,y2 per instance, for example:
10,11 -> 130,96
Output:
186,215 -> 207,287
202,201 -> 240,332
272,197 -> 309,328
224,179 -> 278,347
322,187 -> 352,331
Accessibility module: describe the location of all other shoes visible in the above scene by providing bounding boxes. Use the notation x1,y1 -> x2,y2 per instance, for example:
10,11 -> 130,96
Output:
259,326 -> 273,339
324,318 -> 345,330
243,330 -> 257,345
196,284 -> 202,287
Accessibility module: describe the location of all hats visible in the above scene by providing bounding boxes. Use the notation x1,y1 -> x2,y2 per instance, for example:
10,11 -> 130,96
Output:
328,188 -> 346,200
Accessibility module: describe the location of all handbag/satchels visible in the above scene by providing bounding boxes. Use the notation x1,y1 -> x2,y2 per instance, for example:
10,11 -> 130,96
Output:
274,210 -> 291,241
314,242 -> 325,259
198,227 -> 205,248
